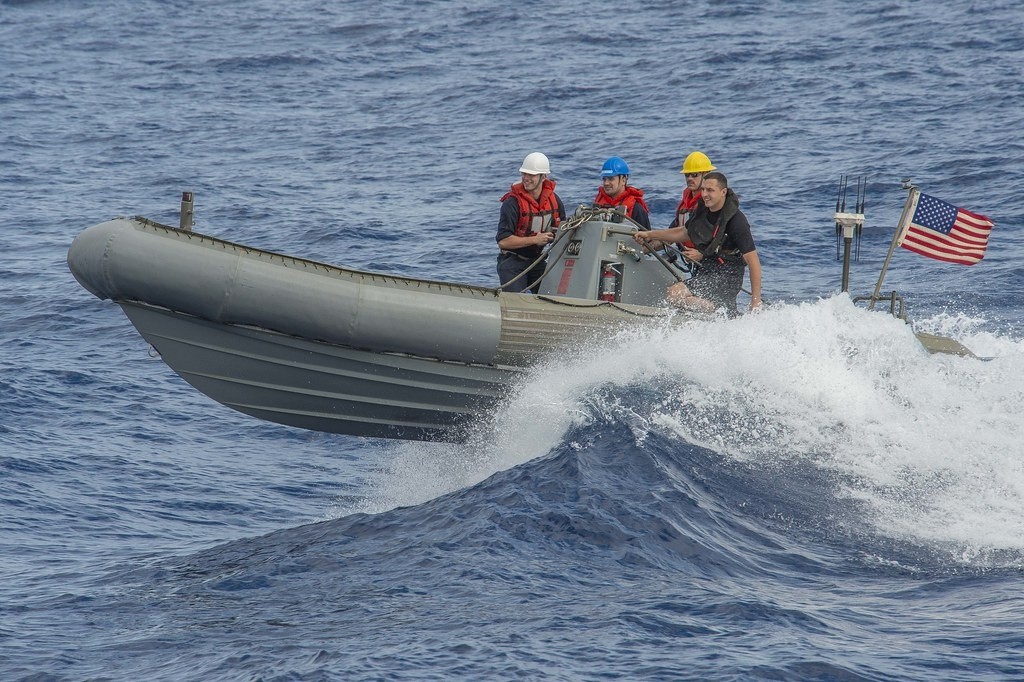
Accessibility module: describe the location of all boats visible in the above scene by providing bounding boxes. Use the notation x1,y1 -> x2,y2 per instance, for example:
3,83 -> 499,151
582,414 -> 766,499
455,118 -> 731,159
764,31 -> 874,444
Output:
67,195 -> 995,441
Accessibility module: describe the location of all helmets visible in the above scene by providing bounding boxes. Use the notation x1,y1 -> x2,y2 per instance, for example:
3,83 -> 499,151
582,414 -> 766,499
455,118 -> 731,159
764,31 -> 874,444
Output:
519,152 -> 551,175
601,157 -> 631,176
680,152 -> 716,173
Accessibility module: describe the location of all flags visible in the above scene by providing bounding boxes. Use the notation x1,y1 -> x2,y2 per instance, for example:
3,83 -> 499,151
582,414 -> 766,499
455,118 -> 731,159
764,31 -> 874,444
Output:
897,191 -> 994,266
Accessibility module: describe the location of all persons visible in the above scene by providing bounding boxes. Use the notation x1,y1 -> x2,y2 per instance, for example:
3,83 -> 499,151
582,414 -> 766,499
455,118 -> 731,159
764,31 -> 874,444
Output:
496,153 -> 565,293
642,152 -> 717,275
632,172 -> 761,316
593,156 -> 652,250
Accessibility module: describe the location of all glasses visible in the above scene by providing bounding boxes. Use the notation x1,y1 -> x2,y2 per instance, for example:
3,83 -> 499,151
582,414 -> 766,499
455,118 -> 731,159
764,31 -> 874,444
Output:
685,172 -> 704,177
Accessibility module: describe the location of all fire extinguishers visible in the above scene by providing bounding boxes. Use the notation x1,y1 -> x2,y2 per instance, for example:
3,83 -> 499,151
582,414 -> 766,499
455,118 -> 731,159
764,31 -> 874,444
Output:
600,261 -> 624,303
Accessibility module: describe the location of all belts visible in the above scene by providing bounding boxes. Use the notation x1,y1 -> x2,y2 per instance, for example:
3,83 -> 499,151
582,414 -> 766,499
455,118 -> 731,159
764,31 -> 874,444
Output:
502,250 -> 532,261
